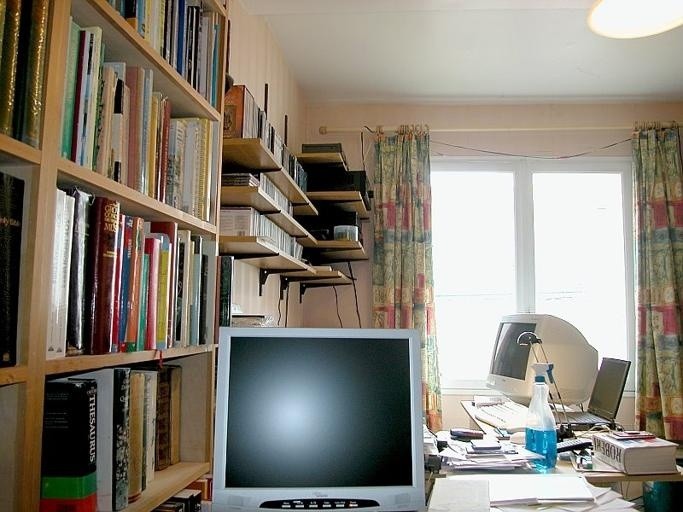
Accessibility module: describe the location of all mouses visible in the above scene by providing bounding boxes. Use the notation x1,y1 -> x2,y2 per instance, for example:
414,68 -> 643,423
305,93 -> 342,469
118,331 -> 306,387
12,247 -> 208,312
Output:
510,432 -> 527,443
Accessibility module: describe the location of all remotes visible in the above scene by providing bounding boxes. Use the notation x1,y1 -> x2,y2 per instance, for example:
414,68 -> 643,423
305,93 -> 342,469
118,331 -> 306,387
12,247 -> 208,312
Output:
556,437 -> 592,453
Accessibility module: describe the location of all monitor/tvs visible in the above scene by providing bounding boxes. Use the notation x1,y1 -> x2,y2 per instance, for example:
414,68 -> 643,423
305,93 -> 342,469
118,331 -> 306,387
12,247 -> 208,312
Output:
211,326 -> 427,512
485,313 -> 599,405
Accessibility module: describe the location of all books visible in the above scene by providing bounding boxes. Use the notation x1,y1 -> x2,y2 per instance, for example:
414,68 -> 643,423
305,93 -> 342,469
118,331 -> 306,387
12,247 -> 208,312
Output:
427,425 -> 683,512
0,0 -> 223,511
224,84 -> 307,261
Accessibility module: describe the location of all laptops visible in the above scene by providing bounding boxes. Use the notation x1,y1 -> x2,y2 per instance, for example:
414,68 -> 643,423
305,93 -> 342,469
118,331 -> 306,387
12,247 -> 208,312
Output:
553,357 -> 632,429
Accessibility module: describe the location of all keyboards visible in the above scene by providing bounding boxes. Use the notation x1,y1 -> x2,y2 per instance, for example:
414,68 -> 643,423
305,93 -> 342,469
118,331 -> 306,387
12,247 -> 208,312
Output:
475,404 -> 532,428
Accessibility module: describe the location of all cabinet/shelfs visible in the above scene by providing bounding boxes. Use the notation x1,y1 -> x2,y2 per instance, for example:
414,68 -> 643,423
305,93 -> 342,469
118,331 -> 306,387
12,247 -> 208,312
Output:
2,3 -> 230,509
224,76 -> 317,285
282,127 -> 373,302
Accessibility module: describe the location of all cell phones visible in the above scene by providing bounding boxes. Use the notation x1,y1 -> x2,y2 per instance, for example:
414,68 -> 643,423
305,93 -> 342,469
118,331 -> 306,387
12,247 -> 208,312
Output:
450,428 -> 482,438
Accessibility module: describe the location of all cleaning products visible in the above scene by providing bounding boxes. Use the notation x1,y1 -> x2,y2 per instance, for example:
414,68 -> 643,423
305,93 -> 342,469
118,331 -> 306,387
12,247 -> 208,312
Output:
525,362 -> 558,471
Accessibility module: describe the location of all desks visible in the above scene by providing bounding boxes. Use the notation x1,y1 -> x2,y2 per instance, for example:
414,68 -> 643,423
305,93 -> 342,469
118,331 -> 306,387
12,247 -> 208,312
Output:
461,400 -> 683,484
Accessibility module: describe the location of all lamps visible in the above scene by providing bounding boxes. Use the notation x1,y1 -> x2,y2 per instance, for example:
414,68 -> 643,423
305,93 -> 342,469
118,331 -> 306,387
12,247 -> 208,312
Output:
517,332 -> 574,443
586,0 -> 683,40
